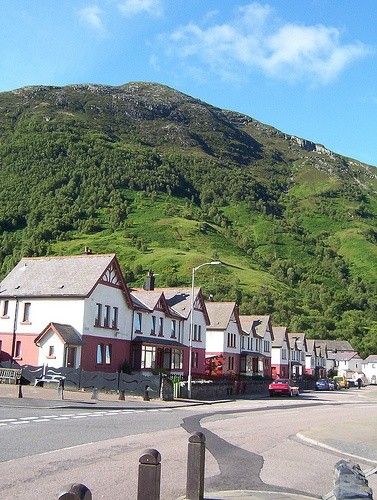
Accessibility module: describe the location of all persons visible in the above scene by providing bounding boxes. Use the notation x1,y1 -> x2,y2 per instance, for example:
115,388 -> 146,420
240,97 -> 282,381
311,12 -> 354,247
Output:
234,379 -> 246,394
356,378 -> 363,389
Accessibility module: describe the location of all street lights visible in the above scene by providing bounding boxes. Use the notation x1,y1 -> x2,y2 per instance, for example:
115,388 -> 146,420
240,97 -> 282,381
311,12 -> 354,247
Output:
188,262 -> 222,398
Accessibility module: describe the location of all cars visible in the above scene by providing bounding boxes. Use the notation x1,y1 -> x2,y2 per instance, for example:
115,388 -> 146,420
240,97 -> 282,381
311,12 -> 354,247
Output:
314,376 -> 349,391
267,380 -> 301,397
